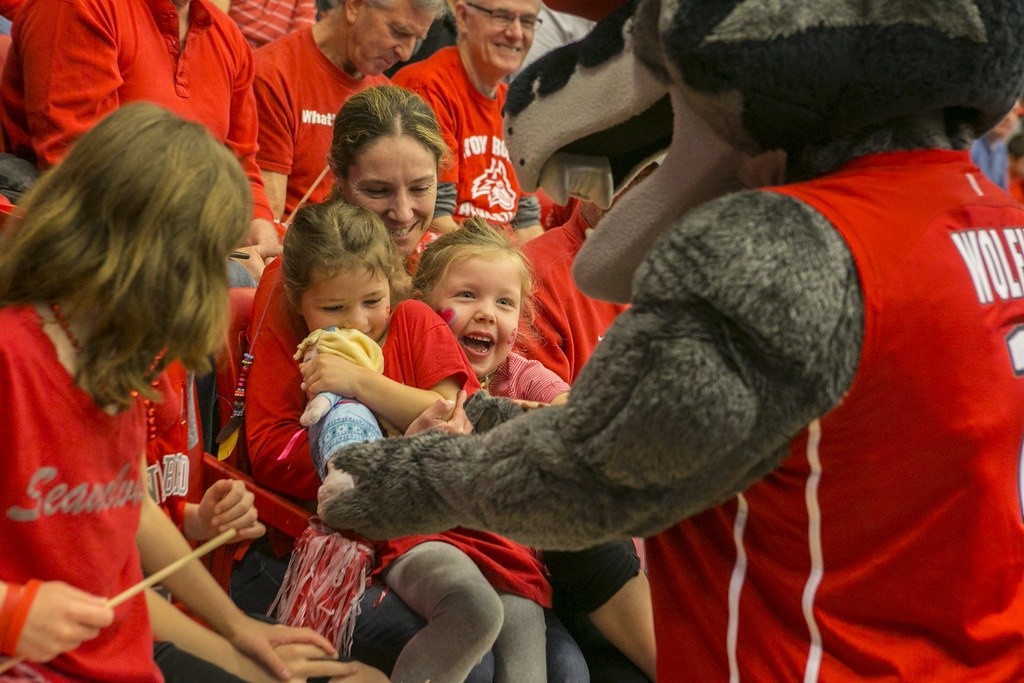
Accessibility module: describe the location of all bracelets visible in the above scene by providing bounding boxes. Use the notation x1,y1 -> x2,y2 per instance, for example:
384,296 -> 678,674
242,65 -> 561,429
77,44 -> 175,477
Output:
0,578 -> 39,657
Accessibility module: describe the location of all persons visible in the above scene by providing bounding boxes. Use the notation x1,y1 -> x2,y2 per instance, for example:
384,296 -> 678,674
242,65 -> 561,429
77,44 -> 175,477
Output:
284,202 -> 552,683
0,0 -> 1023,682
0,102 -> 334,683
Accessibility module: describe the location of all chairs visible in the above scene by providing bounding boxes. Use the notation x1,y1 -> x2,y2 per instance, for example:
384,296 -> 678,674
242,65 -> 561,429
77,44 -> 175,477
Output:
198,284 -> 375,595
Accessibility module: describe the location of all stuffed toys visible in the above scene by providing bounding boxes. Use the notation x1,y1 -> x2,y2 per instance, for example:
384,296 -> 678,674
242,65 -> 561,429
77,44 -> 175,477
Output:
317,1 -> 1024,683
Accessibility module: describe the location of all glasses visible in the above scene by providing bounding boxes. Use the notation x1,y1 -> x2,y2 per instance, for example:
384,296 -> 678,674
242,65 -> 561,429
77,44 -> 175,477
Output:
466,2 -> 543,32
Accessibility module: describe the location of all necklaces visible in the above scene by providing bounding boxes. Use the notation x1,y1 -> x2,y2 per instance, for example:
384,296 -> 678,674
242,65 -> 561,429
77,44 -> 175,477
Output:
50,295 -> 86,353
